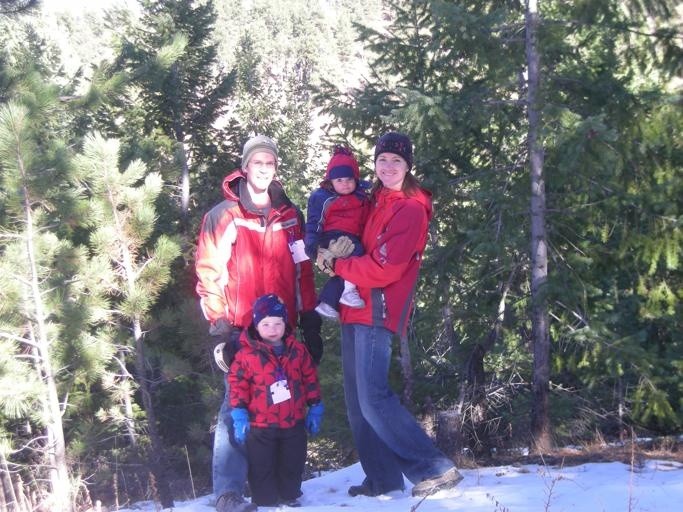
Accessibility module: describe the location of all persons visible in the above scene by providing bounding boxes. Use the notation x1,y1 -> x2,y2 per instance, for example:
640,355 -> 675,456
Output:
230,296 -> 324,508
305,150 -> 370,320
340,132 -> 462,496
195,138 -> 323,511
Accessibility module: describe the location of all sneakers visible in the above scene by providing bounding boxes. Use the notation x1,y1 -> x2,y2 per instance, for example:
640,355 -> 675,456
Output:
315,301 -> 340,319
339,288 -> 366,309
252,497 -> 279,507
411,466 -> 465,498
280,495 -> 302,508
215,491 -> 259,512
347,470 -> 407,499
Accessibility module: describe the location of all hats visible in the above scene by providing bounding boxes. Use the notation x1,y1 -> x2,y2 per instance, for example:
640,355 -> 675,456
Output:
326,145 -> 360,181
242,136 -> 279,171
374,132 -> 413,168
252,292 -> 289,327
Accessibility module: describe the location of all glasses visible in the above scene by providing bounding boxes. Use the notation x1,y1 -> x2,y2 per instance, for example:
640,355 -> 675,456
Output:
249,160 -> 277,169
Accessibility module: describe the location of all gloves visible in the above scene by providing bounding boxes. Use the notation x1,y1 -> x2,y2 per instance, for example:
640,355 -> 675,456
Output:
230,407 -> 251,447
207,315 -> 245,376
315,248 -> 337,274
304,399 -> 325,439
300,309 -> 324,367
327,236 -> 355,260
